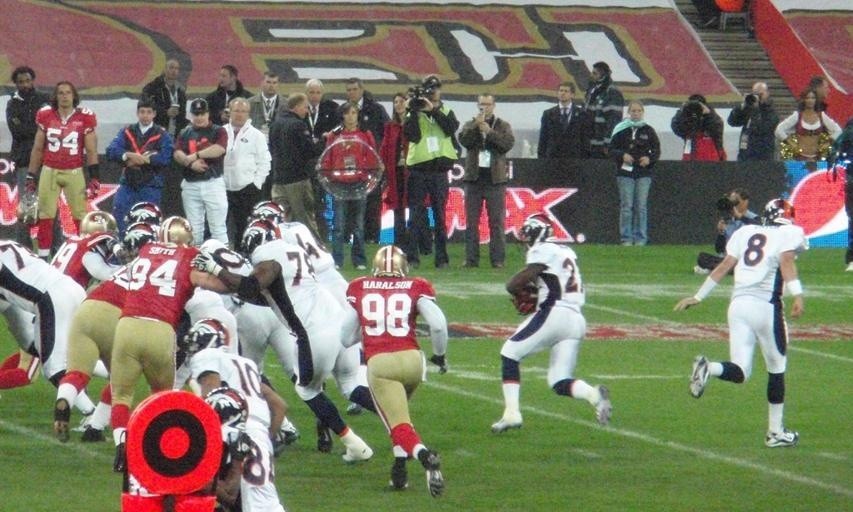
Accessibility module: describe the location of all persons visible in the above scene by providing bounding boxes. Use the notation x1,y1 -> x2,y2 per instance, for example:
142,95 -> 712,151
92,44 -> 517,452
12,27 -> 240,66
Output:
491,213 -> 613,434
315,101 -> 377,271
107,215 -> 235,471
105,99 -> 175,240
334,77 -> 391,244
220,97 -> 273,252
674,198 -> 800,447
834,117 -> 853,273
172,97 -> 227,250
198,238 -> 334,454
247,199 -> 369,416
0,240 -> 97,434
402,74 -> 461,268
203,64 -> 255,127
671,95 -> 727,161
182,318 -> 287,512
774,87 -> 842,165
378,92 -> 434,257
727,82 -> 780,163
191,219 -> 378,463
606,98 -> 661,249
0,209 -> 121,391
693,187 -> 762,277
5,64 -> 54,251
808,75 -> 831,112
24,81 -> 100,263
141,59 -> 190,139
171,286 -> 242,393
244,71 -> 290,131
583,62 -> 624,158
538,79 -> 604,159
267,92 -> 329,249
300,78 -> 339,246
53,256 -> 141,445
458,93 -> 515,269
340,245 -> 447,500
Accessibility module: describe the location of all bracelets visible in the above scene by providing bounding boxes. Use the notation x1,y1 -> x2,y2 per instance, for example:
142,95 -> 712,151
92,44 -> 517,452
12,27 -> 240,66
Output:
194,151 -> 202,161
786,278 -> 804,298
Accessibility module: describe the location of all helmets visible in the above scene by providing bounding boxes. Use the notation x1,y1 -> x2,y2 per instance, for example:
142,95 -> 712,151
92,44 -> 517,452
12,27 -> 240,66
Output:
160,216 -> 193,247
240,219 -> 277,254
521,214 -> 551,248
762,199 -> 791,224
123,222 -> 156,251
202,388 -> 248,432
123,203 -> 161,227
183,318 -> 229,364
79,212 -> 119,238
371,244 -> 410,278
247,200 -> 286,224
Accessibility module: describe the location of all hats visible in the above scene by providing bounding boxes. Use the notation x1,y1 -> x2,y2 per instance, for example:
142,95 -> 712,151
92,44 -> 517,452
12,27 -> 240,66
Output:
191,100 -> 207,114
422,75 -> 441,90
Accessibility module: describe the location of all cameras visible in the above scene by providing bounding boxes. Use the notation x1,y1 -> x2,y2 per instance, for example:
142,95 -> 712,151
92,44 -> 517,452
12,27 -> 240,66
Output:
716,194 -> 741,211
682,101 -> 703,123
744,92 -> 762,105
406,85 -> 433,113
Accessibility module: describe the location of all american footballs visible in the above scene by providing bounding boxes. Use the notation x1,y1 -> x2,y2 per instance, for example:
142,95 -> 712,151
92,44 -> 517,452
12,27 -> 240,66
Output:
512,282 -> 538,315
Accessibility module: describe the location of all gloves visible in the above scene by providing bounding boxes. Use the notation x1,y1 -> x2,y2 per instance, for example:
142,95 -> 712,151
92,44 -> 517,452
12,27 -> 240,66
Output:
429,355 -> 448,375
189,254 -> 223,276
25,175 -> 38,193
87,178 -> 100,197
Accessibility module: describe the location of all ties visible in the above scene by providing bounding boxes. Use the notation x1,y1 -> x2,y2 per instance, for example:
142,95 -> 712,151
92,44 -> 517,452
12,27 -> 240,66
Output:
563,108 -> 567,120
312,107 -> 315,122
266,99 -> 271,113
171,85 -> 175,95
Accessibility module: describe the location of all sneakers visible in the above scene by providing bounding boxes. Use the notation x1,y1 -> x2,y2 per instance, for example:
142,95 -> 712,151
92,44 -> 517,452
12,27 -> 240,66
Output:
694,266 -> 710,273
271,423 -> 300,456
355,265 -> 366,270
345,405 -> 364,415
844,261 -> 853,272
412,263 -> 418,269
342,444 -> 372,464
494,262 -> 503,268
765,428 -> 798,448
422,450 -> 443,497
113,444 -> 125,471
436,262 -> 448,270
490,411 -> 522,434
71,407 -> 97,432
53,397 -> 69,444
80,426 -> 110,444
316,421 -> 333,453
390,468 -> 408,489
593,384 -> 612,425
689,355 -> 709,397
